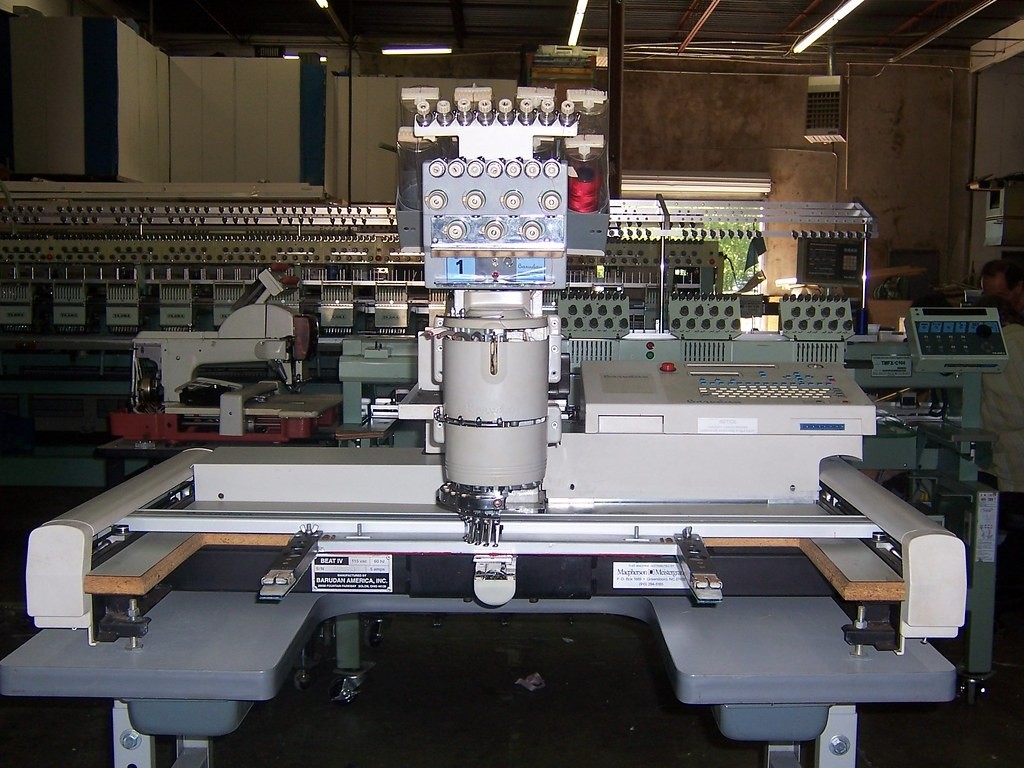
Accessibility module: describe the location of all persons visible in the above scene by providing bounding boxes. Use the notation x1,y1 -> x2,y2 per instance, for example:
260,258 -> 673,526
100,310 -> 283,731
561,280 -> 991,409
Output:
979,249 -> 1024,632
